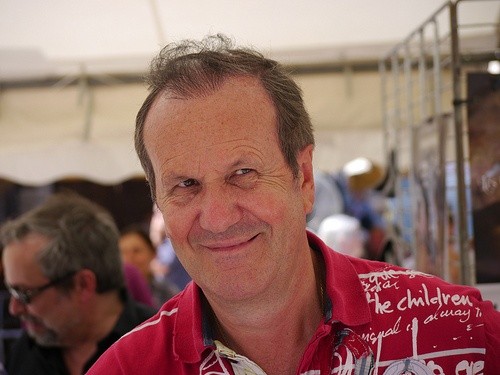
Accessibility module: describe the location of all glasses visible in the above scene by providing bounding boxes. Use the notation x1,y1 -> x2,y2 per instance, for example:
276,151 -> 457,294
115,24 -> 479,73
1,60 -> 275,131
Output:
5,267 -> 76,304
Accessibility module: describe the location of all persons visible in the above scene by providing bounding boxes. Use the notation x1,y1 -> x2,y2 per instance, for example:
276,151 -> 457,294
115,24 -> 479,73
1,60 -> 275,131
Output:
0,159 -> 406,375
85,34 -> 500,375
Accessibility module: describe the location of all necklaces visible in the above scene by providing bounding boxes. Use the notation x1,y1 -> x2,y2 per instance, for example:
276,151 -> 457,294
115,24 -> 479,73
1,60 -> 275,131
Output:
319,270 -> 325,313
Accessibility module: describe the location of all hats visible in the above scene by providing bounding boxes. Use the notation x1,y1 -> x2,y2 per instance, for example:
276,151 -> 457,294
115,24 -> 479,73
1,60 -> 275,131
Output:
315,213 -> 366,259
